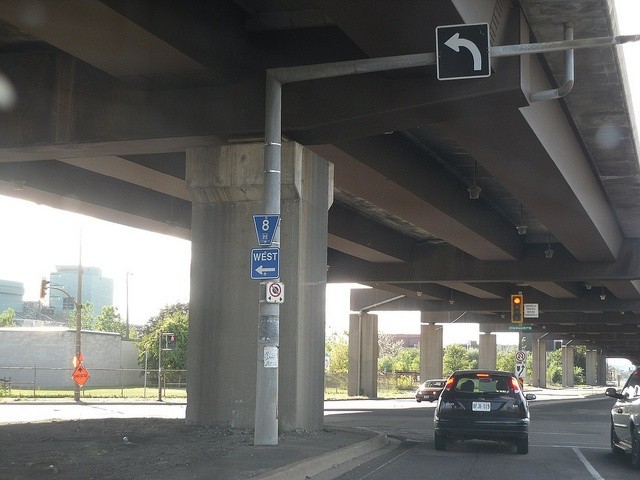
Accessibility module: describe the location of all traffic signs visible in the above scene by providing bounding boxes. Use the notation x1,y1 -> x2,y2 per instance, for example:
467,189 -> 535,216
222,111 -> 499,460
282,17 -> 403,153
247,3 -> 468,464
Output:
71,362 -> 90,388
249,248 -> 280,280
253,213 -> 280,246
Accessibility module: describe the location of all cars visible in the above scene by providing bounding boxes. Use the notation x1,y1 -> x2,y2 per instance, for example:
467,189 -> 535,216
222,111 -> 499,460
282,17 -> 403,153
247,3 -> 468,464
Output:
433,369 -> 537,455
605,367 -> 640,469
415,379 -> 448,403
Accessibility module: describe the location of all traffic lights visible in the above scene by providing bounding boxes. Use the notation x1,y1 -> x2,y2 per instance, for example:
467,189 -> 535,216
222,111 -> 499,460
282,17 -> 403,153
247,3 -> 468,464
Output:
511,294 -> 523,324
41,279 -> 50,298
